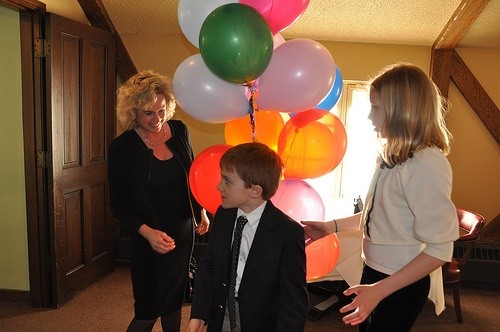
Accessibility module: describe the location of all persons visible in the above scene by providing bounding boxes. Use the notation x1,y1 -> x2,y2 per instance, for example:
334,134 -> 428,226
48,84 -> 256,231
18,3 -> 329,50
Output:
107,71 -> 210,332
301,62 -> 460,332
186,142 -> 309,332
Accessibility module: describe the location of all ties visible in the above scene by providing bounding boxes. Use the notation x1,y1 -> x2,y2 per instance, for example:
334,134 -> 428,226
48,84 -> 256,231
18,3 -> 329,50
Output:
227,216 -> 248,332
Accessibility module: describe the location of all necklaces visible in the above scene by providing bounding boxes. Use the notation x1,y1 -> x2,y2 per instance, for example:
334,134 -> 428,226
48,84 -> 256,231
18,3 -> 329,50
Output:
141,124 -> 166,146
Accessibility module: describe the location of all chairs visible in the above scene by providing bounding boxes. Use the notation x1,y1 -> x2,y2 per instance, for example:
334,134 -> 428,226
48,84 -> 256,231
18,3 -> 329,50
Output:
441,208 -> 485,324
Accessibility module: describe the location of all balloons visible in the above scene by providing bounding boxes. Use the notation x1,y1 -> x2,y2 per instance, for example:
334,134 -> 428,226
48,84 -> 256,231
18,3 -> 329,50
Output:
171,0 -> 347,280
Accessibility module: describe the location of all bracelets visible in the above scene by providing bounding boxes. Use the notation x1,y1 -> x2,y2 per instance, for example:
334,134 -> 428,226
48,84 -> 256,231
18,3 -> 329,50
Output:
333,219 -> 338,232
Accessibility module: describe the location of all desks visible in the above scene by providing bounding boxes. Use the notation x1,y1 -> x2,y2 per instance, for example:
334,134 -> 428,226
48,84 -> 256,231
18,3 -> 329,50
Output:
305,230 -> 446,317
